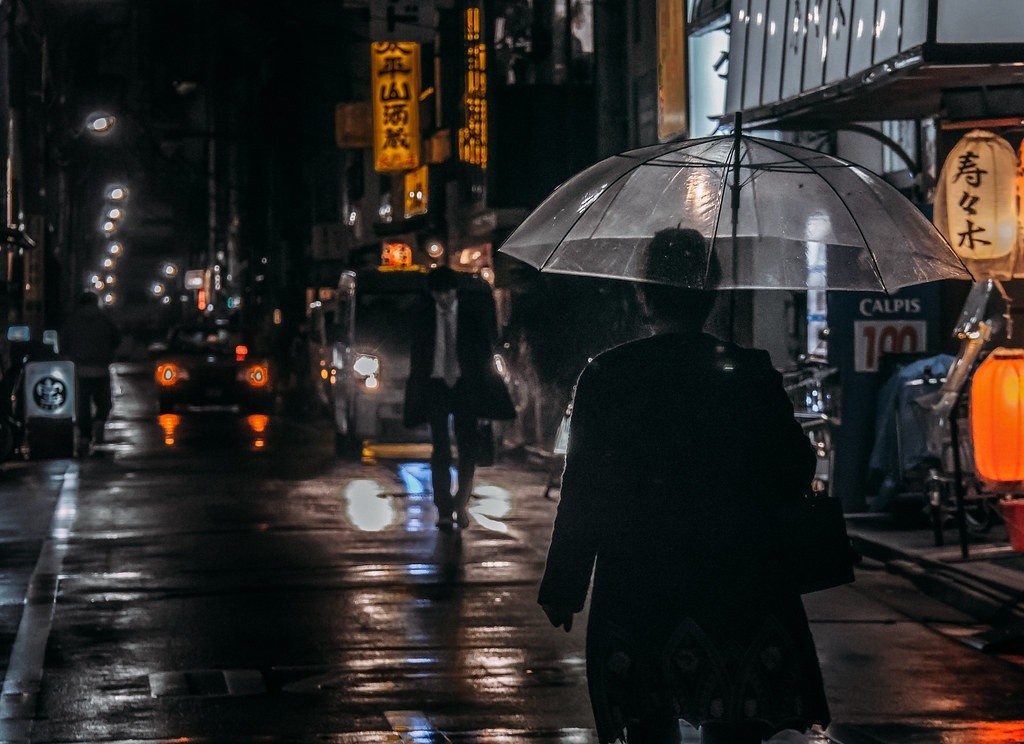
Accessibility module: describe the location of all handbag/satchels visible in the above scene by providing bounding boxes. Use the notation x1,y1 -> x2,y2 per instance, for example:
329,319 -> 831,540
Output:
784,485 -> 859,592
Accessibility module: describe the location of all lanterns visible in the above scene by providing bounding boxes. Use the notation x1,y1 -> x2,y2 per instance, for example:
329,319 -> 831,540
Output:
970,342 -> 1024,489
928,131 -> 1016,281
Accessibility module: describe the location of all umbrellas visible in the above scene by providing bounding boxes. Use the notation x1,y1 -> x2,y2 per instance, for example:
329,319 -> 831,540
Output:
496,110 -> 977,345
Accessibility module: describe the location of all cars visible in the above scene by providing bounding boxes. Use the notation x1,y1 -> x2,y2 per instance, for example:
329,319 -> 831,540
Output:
303,301 -> 332,406
152,317 -> 271,415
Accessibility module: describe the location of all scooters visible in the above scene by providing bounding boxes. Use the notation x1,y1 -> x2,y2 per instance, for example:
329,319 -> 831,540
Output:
891,352 -> 1001,534
776,362 -> 842,496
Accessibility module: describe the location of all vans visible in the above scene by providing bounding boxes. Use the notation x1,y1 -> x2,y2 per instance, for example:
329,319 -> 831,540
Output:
331,266 -> 496,467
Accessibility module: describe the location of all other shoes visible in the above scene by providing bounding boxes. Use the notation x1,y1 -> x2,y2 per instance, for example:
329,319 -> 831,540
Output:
434,514 -> 453,530
454,493 -> 470,529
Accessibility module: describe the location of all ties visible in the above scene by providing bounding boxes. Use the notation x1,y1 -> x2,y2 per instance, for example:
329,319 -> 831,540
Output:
442,310 -> 457,388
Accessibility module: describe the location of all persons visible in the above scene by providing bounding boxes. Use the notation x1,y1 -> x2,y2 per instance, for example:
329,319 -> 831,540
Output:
56,292 -> 120,454
538,229 -> 833,744
400,266 -> 518,527
